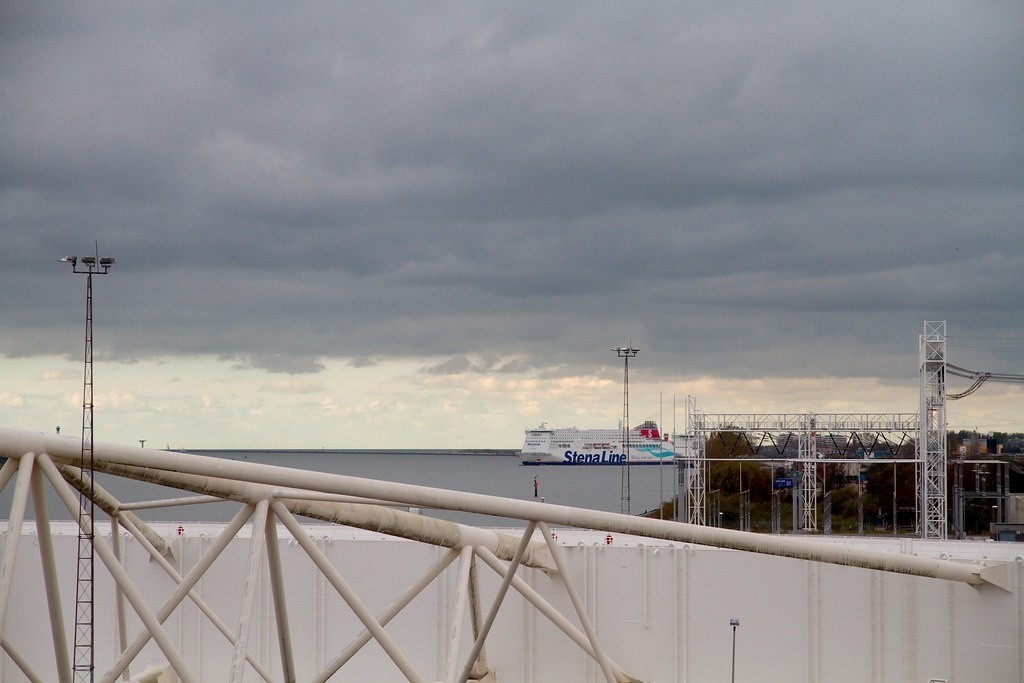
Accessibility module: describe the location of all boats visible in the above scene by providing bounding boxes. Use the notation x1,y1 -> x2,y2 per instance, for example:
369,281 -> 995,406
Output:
514,420 -> 677,465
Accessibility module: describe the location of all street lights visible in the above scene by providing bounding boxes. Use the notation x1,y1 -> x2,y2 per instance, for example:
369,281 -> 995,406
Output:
59,255 -> 115,683
612,346 -> 640,514
